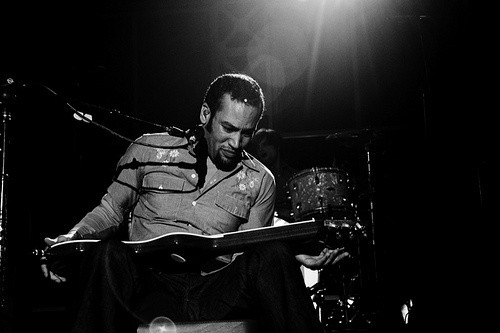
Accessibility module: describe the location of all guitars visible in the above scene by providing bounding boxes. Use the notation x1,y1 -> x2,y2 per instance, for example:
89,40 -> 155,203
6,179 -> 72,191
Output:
44,215 -> 368,274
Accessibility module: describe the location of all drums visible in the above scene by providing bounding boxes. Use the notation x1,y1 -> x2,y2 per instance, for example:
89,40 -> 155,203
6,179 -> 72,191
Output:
286,167 -> 360,221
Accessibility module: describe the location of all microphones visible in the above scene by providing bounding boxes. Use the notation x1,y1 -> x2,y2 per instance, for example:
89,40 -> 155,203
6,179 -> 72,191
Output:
187,125 -> 205,145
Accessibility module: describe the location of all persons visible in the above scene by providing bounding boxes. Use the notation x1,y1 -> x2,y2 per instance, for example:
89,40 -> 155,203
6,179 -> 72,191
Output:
253,128 -> 294,219
40,73 -> 349,284
